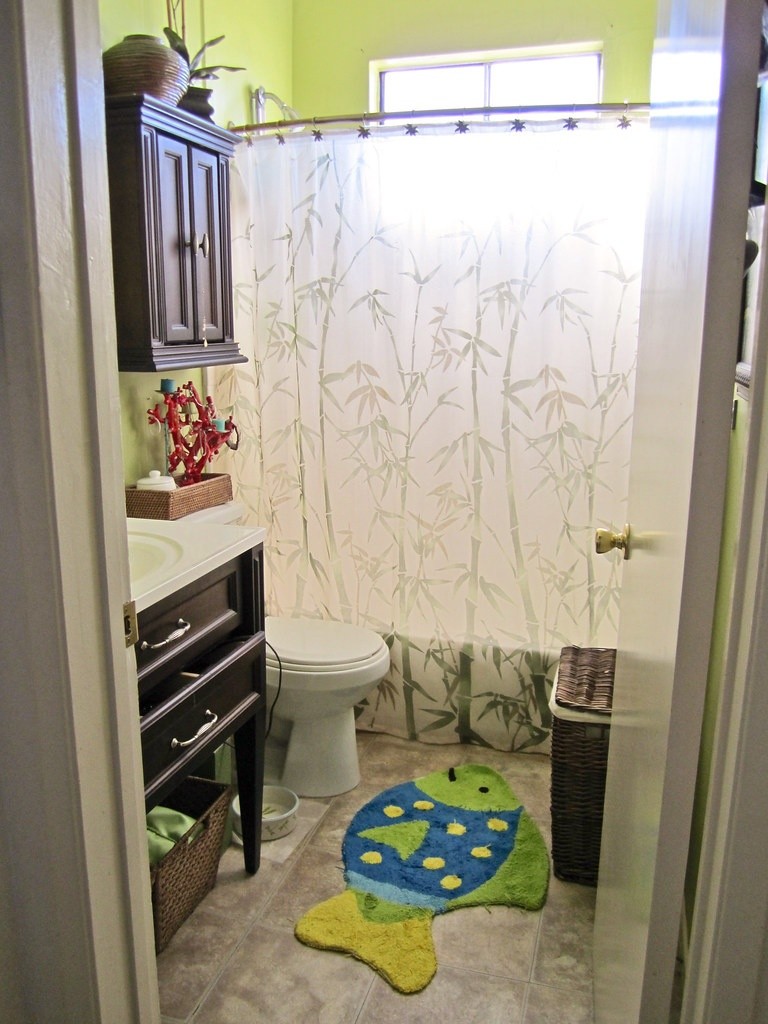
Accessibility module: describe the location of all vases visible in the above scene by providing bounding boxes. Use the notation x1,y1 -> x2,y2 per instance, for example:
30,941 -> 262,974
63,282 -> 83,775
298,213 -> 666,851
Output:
103,32 -> 190,107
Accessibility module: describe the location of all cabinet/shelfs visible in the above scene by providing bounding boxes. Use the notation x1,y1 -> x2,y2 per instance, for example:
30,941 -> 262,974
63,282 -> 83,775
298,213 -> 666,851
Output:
106,91 -> 249,376
128,541 -> 268,878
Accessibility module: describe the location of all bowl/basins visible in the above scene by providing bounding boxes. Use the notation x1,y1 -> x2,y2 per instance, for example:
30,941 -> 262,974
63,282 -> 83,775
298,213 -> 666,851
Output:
230,785 -> 300,841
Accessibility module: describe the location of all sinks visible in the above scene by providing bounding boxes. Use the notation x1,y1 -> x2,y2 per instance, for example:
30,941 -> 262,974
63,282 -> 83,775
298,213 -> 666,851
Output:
127,530 -> 183,592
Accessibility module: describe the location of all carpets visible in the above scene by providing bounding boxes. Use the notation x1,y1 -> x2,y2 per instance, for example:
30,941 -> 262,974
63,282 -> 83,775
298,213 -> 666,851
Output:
293,763 -> 550,993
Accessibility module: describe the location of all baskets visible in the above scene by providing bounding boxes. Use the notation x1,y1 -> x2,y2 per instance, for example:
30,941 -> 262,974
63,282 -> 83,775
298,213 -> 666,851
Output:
149,776 -> 232,956
126,473 -> 233,520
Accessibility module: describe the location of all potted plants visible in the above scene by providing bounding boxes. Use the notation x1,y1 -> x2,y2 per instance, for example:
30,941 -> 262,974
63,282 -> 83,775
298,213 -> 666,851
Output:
162,27 -> 247,120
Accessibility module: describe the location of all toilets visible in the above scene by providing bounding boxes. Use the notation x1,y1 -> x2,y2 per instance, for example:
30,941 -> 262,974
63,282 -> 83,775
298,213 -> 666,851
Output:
265,612 -> 390,798
